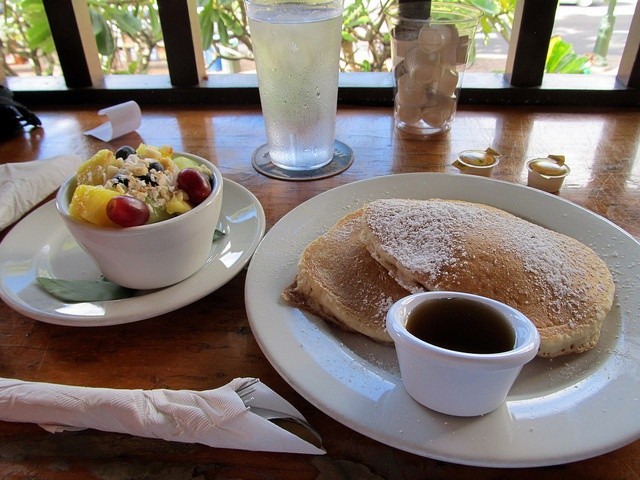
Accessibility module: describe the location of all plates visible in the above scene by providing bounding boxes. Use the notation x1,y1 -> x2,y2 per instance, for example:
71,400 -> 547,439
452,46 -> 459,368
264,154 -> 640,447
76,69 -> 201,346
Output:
245,171 -> 640,468
0,179 -> 266,328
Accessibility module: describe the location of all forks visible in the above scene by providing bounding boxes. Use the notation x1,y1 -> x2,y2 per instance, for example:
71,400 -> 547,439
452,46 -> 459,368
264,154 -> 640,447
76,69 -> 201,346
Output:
235,377 -> 260,414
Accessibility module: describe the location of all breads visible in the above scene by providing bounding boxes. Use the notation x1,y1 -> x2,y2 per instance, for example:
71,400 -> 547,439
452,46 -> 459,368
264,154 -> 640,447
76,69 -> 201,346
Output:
281,206 -> 411,345
360,198 -> 616,357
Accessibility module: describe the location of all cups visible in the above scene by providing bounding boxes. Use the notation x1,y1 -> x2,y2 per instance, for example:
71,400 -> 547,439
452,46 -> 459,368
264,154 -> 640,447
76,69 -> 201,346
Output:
384,4 -> 484,137
244,0 -> 345,170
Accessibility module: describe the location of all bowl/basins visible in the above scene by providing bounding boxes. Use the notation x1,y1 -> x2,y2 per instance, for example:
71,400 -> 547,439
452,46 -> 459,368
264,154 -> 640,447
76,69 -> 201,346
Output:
54,152 -> 222,293
385,291 -> 540,418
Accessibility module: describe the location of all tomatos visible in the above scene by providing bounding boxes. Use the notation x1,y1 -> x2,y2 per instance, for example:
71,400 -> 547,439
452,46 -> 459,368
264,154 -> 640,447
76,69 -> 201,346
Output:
106,196 -> 149,227
177,168 -> 212,201
